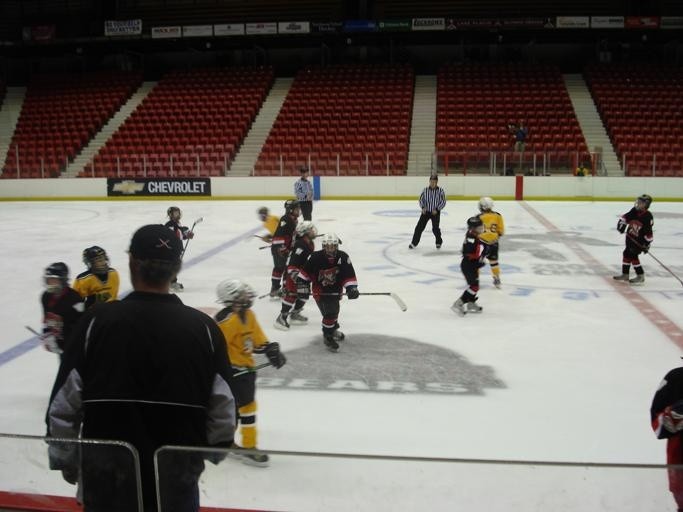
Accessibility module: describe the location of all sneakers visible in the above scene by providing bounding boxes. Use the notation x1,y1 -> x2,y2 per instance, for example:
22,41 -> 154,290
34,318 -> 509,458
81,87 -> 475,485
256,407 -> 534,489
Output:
467,301 -> 484,314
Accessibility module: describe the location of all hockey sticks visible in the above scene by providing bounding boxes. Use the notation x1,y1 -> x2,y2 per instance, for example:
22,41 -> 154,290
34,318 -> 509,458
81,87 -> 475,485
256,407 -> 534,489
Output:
180,216 -> 204,263
259,234 -> 343,250
309,292 -> 407,312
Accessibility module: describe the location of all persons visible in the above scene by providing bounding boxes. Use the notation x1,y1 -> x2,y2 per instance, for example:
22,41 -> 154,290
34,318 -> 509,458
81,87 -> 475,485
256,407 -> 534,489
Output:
473,198 -> 504,288
269,200 -> 301,301
513,122 -> 528,152
451,217 -> 484,317
294,167 -> 313,221
41,262 -> 82,356
297,233 -> 358,352
273,222 -> 317,331
255,208 -> 279,243
164,207 -> 194,292
506,165 -> 514,176
650,367 -> 683,512
212,281 -> 286,468
574,162 -> 589,177
44,224 -> 239,512
74,246 -> 120,306
408,174 -> 446,250
613,194 -> 654,285
524,168 -> 540,176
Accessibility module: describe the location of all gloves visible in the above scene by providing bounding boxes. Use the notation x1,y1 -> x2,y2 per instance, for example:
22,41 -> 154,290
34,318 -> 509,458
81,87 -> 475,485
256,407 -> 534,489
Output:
616,218 -> 626,234
295,280 -> 311,299
346,284 -> 359,298
266,341 -> 286,370
278,245 -> 288,257
182,230 -> 194,240
475,258 -> 486,269
41,333 -> 64,356
262,235 -> 272,243
640,240 -> 651,254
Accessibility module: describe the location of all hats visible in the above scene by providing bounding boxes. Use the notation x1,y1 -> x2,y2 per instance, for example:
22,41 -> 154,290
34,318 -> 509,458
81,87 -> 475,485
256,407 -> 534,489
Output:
126,223 -> 185,264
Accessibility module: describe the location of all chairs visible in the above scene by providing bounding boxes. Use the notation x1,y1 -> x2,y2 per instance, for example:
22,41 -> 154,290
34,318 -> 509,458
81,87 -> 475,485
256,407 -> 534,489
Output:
248,65 -> 414,176
2,66 -> 144,178
75,65 -> 276,177
436,65 -> 593,173
581,67 -> 683,178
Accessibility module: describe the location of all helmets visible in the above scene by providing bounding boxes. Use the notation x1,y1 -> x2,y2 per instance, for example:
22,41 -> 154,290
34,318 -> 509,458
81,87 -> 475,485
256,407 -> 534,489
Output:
40,261 -> 69,294
216,279 -> 258,308
467,217 -> 482,228
81,246 -> 110,264
478,195 -> 494,211
321,233 -> 342,247
257,207 -> 269,222
284,198 -> 300,212
167,206 -> 181,216
634,193 -> 652,214
296,220 -> 314,236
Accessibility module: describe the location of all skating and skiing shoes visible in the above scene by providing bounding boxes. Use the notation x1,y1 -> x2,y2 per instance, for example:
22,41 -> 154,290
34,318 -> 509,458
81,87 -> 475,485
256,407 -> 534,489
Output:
629,273 -> 645,286
323,338 -> 341,354
334,328 -> 345,342
169,281 -> 184,295
274,315 -> 289,331
228,445 -> 242,460
435,243 -> 441,251
493,275 -> 502,289
613,274 -> 629,283
288,311 -> 309,326
450,298 -> 464,319
409,241 -> 418,249
241,444 -> 268,469
269,289 -> 285,298
